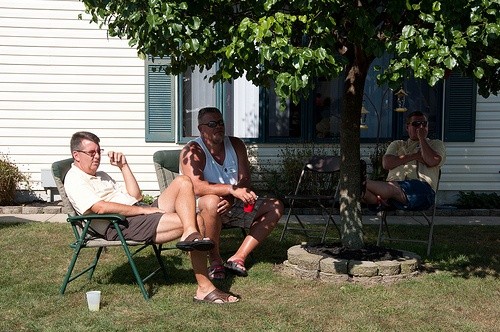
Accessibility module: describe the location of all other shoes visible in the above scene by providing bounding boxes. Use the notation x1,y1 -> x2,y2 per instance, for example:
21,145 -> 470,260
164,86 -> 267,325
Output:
359,159 -> 367,200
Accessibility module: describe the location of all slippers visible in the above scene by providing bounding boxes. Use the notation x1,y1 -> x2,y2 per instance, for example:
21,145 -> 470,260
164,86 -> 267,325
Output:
192,288 -> 240,303
224,260 -> 248,277
208,265 -> 227,279
176,232 -> 215,251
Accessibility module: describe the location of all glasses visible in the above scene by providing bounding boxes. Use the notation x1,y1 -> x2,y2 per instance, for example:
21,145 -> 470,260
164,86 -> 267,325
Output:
200,120 -> 225,128
74,148 -> 105,157
410,120 -> 429,127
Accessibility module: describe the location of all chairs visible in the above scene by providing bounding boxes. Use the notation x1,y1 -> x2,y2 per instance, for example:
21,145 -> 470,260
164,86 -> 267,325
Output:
153,150 -> 257,265
278,155 -> 342,244
375,167 -> 440,257
51,157 -> 171,302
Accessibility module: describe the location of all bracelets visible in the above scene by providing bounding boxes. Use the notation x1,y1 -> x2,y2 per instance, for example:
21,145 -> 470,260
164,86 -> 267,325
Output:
122,163 -> 127,165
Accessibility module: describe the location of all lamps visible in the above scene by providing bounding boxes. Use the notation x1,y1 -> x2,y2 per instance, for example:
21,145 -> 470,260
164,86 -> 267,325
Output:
393,87 -> 408,112
361,105 -> 369,129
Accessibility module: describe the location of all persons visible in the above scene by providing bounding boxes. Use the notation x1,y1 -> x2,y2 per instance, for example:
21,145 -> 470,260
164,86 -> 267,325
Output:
176,108 -> 284,280
360,111 -> 446,212
64,132 -> 241,304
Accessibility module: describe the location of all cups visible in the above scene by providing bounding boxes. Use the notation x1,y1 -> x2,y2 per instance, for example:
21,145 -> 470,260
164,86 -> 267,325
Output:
244,196 -> 258,213
86,290 -> 101,311
